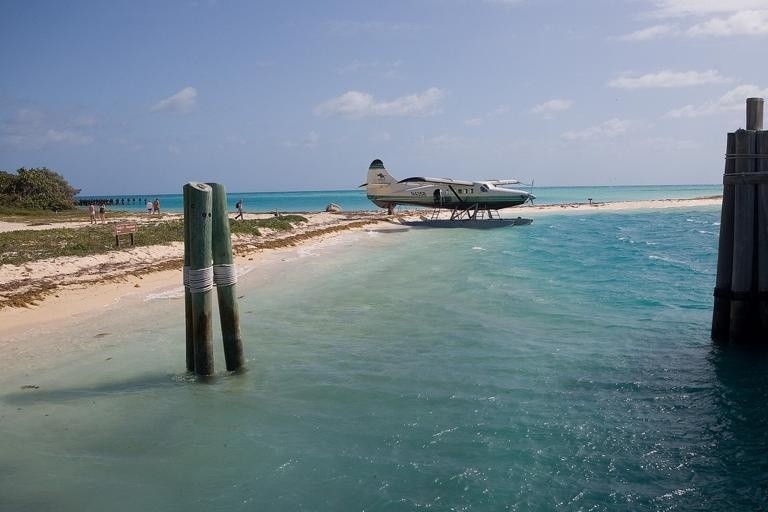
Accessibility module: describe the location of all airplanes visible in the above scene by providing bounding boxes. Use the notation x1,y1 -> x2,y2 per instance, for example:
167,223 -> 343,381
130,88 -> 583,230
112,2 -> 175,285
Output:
366,157 -> 538,211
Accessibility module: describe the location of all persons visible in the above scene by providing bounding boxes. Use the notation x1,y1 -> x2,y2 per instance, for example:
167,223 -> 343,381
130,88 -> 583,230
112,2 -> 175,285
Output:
88,202 -> 97,224
146,200 -> 153,217
100,202 -> 106,224
234,198 -> 245,220
152,198 -> 162,217
